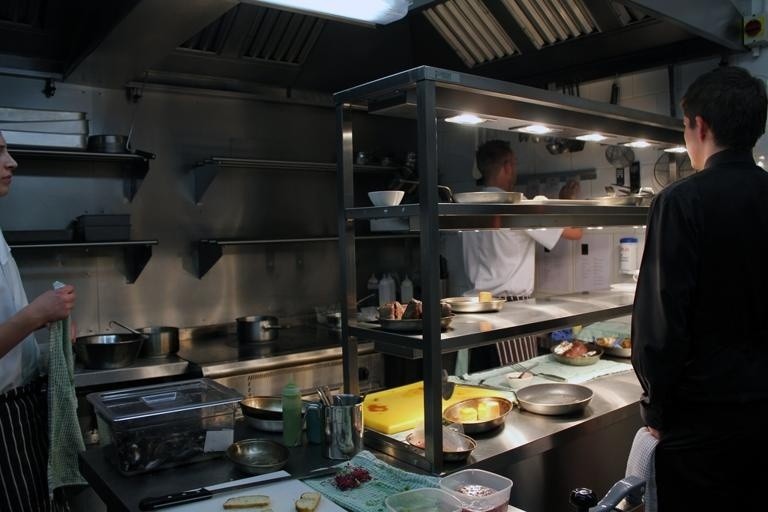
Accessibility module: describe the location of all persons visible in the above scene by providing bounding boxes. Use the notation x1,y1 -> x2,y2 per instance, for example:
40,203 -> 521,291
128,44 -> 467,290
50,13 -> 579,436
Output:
630,66 -> 768,512
1,132 -> 81,512
461,137 -> 583,375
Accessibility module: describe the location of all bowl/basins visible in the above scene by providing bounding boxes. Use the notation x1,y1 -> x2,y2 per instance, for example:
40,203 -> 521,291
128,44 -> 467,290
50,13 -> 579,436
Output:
87,132 -> 130,156
368,187 -> 404,208
225,437 -> 292,473
548,343 -> 606,366
407,383 -> 594,463
71,334 -> 144,370
384,468 -> 514,511
238,393 -> 307,432
505,371 -> 533,389
374,296 -> 507,330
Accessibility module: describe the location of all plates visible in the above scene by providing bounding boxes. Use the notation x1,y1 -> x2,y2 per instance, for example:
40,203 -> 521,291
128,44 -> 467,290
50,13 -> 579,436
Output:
452,189 -> 643,204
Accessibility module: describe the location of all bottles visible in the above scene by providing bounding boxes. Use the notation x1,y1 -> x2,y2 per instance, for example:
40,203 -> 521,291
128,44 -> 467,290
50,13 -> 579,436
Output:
618,237 -> 639,273
281,370 -> 304,447
367,269 -> 414,309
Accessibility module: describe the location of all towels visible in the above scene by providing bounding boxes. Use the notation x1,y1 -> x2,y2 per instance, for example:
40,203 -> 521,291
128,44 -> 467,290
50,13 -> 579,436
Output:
301,449 -> 459,512
613,427 -> 660,512
46,280 -> 89,503
462,353 -> 634,404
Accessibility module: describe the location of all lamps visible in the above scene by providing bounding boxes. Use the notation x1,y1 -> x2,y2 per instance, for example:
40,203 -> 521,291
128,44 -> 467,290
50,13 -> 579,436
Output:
241,0 -> 414,29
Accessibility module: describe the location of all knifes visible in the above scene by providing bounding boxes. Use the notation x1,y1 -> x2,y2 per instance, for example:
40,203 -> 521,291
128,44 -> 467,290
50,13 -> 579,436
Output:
138,466 -> 342,510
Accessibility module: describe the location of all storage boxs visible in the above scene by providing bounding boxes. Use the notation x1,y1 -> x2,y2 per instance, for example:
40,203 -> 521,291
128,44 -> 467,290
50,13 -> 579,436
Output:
439,468 -> 513,512
384,488 -> 463,512
86,376 -> 248,476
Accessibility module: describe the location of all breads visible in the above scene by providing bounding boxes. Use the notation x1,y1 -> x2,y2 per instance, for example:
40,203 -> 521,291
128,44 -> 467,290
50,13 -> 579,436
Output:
222,493 -> 272,509
295,492 -> 321,512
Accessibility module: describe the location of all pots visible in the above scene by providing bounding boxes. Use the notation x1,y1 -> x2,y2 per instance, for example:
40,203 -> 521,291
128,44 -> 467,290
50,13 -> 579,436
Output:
108,320 -> 180,360
234,312 -> 291,343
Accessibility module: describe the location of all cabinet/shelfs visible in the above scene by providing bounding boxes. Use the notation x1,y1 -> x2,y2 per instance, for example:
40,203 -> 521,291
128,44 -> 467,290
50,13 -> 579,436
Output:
332,66 -> 686,512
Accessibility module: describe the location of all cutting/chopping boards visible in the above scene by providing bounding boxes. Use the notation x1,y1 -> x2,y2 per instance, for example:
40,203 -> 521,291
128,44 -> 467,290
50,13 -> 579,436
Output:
156,469 -> 349,512
351,377 -> 514,434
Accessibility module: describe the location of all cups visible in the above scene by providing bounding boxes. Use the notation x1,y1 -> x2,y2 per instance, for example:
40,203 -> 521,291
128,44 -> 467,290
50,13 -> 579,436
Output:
321,395 -> 365,461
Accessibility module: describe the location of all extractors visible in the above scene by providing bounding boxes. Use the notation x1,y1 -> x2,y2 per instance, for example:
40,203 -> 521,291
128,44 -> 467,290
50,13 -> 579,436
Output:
2,0 -> 738,109
